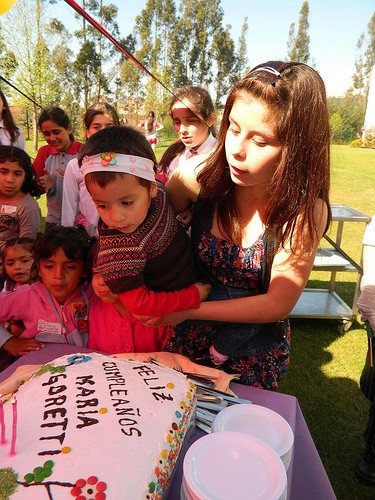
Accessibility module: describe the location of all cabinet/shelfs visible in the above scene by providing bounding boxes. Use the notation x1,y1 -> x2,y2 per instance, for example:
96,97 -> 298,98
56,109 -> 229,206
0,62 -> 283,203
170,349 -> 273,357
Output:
286,204 -> 372,335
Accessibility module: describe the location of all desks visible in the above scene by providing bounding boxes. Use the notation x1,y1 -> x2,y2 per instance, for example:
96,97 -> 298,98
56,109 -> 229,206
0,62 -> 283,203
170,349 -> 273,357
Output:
0,342 -> 337,500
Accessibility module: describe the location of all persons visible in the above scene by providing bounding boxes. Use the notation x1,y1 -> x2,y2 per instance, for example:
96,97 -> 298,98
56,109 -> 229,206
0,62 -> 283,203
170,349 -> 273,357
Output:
355,213 -> 374,485
0,59 -> 333,391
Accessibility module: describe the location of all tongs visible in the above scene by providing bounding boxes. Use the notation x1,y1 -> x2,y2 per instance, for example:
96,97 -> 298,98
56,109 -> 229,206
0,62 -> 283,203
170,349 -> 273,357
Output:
147,357 -> 254,433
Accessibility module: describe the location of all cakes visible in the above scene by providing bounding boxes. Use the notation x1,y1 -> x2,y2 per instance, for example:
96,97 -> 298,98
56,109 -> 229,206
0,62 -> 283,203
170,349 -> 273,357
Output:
0,351 -> 197,500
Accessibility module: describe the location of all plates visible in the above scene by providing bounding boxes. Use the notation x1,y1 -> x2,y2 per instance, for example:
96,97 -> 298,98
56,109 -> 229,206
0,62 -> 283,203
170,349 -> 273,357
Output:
180,431 -> 287,500
212,403 -> 294,473
